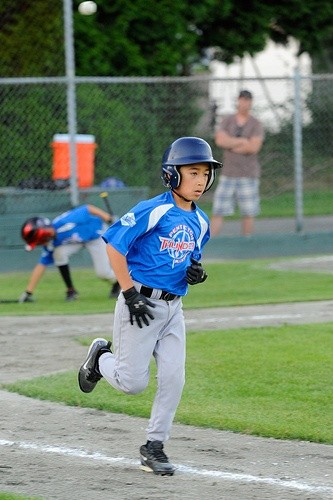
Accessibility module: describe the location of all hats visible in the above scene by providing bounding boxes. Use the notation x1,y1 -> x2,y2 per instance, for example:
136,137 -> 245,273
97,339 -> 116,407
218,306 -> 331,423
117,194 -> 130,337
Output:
240,90 -> 252,99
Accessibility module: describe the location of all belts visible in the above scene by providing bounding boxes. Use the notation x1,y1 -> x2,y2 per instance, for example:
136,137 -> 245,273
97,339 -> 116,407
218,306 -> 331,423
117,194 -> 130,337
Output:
140,285 -> 181,302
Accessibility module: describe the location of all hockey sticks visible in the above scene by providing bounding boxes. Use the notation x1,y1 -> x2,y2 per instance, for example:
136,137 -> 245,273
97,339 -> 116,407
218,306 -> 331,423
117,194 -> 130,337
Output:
101,193 -> 113,215
0,299 -> 34,304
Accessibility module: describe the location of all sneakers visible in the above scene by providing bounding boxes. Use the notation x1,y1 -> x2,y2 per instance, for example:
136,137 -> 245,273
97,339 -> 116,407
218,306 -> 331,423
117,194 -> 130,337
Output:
78,338 -> 112,393
140,441 -> 175,474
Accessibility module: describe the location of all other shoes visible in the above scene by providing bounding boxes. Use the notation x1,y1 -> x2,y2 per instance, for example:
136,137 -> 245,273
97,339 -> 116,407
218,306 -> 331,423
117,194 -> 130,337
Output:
66,290 -> 79,302
108,281 -> 120,299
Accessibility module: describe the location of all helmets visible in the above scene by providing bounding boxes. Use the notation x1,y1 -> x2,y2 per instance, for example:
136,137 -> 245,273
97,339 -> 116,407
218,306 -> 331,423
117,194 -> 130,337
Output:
162,136 -> 223,192
21,217 -> 55,250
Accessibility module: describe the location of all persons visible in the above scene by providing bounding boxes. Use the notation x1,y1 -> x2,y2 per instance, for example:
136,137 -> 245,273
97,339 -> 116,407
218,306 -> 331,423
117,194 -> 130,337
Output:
209,90 -> 266,238
78,137 -> 223,476
19,204 -> 121,303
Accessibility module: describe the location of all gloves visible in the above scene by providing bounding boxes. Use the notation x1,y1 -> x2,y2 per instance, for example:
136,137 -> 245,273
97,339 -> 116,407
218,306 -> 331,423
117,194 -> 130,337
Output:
122,286 -> 155,328
19,292 -> 33,303
184,258 -> 208,285
